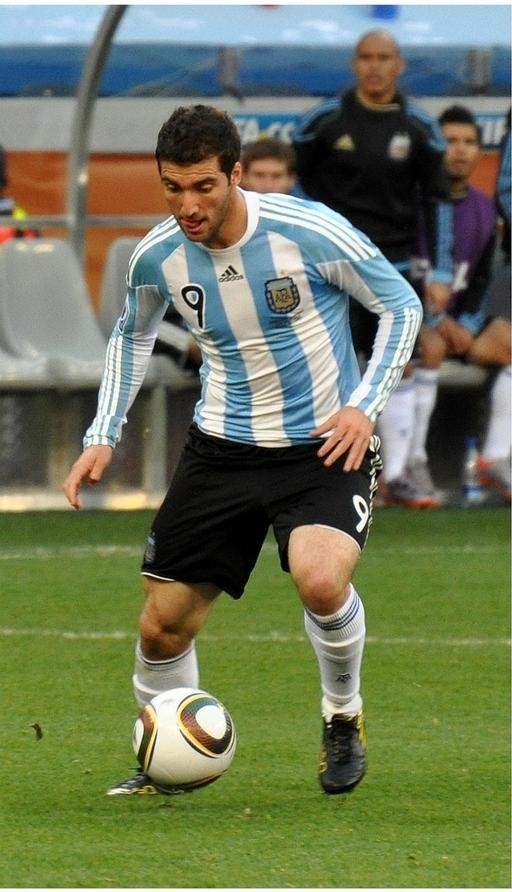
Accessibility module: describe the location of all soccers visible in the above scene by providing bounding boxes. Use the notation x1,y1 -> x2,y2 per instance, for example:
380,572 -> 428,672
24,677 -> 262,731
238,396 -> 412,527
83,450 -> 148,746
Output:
133,688 -> 235,792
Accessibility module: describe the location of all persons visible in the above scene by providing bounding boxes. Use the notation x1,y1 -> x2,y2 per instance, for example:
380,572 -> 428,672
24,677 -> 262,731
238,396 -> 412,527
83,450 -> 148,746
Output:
238,30 -> 512,511
62,107 -> 423,795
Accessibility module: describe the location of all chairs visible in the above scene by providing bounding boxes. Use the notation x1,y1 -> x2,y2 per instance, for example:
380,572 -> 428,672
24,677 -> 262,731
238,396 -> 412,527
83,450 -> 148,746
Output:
2,237 -> 200,495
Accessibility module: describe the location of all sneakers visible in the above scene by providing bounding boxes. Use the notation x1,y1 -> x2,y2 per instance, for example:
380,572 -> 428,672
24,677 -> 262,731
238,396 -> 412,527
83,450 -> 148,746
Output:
376,450 -> 512,508
107,770 -> 158,796
320,706 -> 368,795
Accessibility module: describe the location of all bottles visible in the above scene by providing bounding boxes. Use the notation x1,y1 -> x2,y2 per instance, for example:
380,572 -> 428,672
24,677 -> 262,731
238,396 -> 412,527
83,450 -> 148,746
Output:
463,438 -> 484,505
